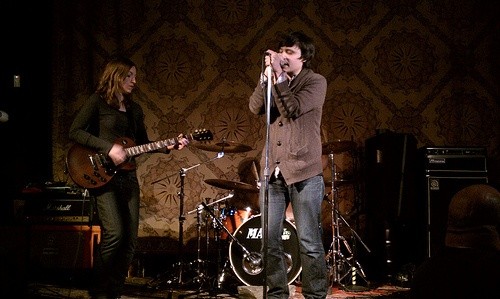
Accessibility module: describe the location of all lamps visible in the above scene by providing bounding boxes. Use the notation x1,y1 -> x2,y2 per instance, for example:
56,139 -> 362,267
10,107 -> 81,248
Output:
0,111 -> 9,122
14,74 -> 21,88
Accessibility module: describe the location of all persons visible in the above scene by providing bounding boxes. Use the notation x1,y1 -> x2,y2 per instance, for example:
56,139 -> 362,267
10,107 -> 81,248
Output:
69,59 -> 190,299
249,32 -> 329,299
410,184 -> 500,299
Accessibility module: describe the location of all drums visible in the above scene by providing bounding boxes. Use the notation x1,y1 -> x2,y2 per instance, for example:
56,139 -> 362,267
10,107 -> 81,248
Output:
228,213 -> 303,287
220,205 -> 254,243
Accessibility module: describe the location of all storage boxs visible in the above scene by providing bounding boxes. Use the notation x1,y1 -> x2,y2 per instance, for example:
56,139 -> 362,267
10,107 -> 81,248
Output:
37,187 -> 92,223
404,146 -> 491,260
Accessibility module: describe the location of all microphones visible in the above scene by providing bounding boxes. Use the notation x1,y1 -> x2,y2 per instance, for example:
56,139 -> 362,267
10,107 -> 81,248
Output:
323,192 -> 331,200
246,254 -> 263,269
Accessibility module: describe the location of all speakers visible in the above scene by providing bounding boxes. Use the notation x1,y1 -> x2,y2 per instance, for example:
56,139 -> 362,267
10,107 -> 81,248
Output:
424,174 -> 489,258
29,181 -> 102,225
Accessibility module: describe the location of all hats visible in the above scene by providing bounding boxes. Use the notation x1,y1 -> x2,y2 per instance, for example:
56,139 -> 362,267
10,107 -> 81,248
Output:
444,184 -> 500,248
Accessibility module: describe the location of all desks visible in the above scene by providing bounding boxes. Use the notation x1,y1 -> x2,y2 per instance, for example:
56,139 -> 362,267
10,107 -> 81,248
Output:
27,224 -> 102,270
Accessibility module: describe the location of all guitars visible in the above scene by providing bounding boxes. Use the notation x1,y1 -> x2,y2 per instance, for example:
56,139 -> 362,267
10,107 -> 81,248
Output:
66,127 -> 215,189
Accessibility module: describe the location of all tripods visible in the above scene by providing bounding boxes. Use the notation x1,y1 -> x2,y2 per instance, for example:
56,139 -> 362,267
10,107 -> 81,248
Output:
318,151 -> 374,291
151,144 -> 245,299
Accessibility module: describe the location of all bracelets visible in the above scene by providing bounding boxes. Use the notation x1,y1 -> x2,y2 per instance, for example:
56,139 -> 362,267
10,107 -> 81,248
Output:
264,74 -> 268,79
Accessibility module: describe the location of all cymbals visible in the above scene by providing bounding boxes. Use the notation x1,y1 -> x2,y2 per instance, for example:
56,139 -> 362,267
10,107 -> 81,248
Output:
324,179 -> 359,186
322,139 -> 358,155
195,139 -> 253,153
204,178 -> 260,193
